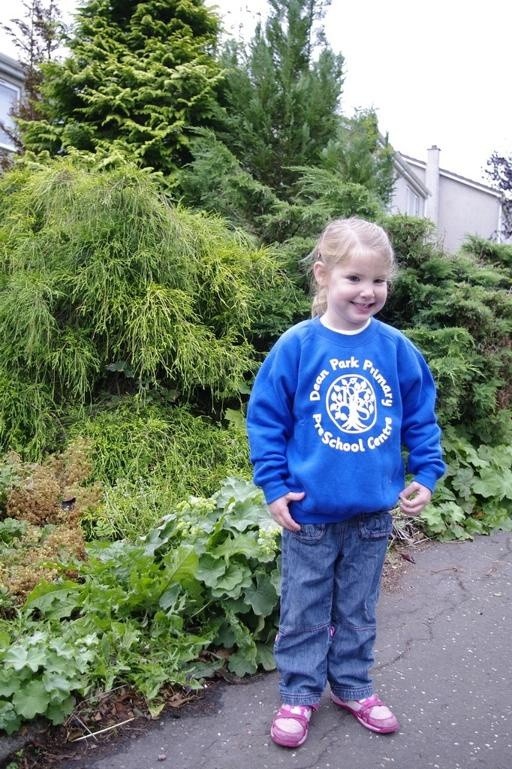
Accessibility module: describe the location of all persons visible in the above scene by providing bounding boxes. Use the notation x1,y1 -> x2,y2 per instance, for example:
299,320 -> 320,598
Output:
245,218 -> 449,750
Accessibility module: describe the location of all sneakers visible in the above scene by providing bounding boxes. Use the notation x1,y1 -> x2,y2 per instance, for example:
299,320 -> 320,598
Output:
330,691 -> 399,734
271,700 -> 321,747
